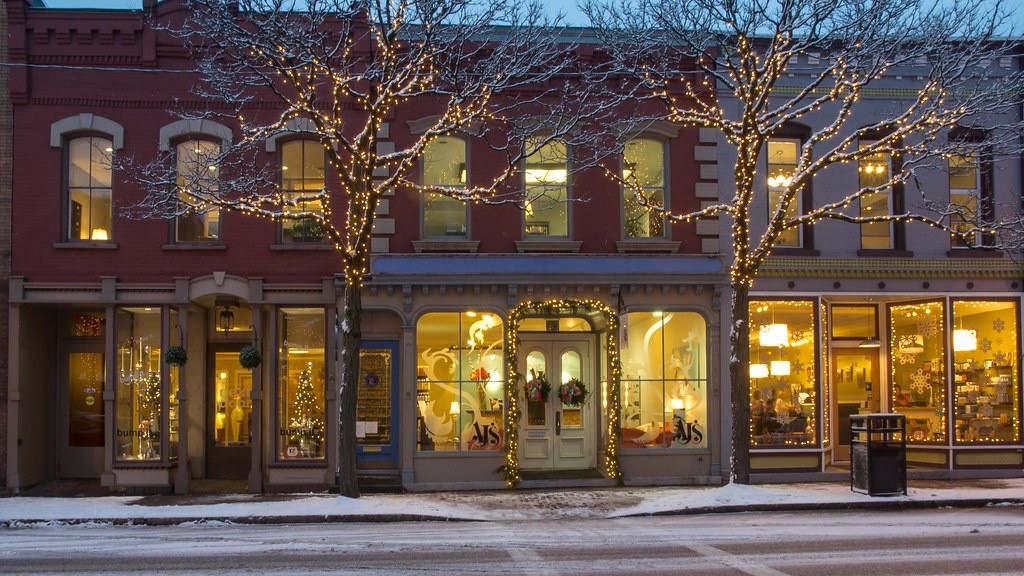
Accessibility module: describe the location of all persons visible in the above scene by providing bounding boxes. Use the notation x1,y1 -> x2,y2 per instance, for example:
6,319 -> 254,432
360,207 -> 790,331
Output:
775,391 -> 790,414
750,390 -> 766,436
894,383 -> 913,406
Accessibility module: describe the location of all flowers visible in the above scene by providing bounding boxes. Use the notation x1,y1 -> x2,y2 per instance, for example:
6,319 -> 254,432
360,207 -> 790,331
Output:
470,367 -> 490,408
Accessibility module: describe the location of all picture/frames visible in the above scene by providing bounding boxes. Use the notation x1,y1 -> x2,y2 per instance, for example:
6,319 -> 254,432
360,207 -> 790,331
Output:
922,361 -> 932,372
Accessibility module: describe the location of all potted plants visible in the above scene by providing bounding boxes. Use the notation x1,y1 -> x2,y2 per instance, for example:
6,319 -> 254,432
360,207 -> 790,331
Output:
239,346 -> 262,370
164,346 -> 188,369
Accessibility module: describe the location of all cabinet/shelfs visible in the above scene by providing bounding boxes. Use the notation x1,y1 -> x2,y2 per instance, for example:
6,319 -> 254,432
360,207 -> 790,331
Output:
621,375 -> 642,426
932,362 -> 1013,439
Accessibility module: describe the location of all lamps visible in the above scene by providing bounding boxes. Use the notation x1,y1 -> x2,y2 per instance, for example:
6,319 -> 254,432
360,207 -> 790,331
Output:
458,162 -> 636,182
899,340 -> 926,350
953,312 -> 976,351
220,306 -> 235,336
767,151 -> 793,188
865,153 -> 883,173
448,401 -> 460,449
92,228 -> 107,240
748,303 -> 791,378
857,294 -> 881,347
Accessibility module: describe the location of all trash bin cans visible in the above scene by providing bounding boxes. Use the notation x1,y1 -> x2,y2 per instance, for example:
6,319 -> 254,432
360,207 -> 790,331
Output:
849,413 -> 908,497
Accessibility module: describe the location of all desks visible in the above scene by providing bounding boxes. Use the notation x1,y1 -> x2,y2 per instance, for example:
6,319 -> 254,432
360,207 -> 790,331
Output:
857,407 -> 936,440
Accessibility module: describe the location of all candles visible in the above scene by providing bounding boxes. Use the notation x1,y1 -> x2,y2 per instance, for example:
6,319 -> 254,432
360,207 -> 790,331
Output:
121,337 -> 160,372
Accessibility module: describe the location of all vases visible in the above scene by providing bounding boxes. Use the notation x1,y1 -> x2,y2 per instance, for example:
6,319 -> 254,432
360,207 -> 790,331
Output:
476,382 -> 487,411
